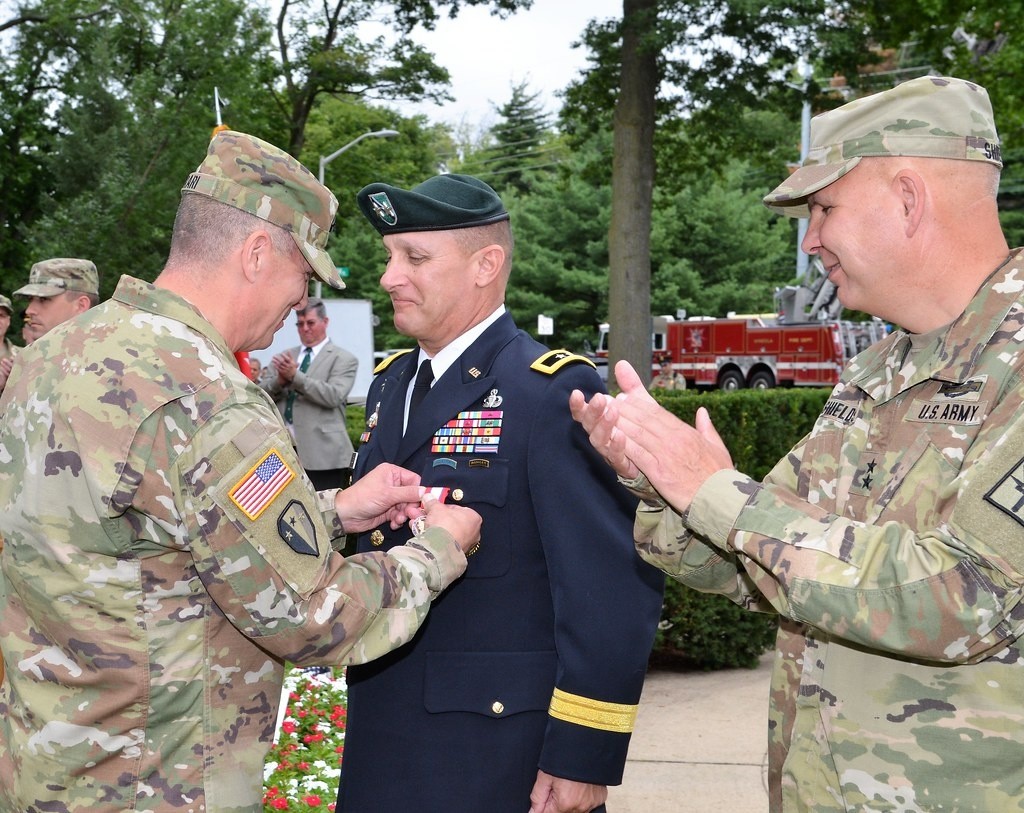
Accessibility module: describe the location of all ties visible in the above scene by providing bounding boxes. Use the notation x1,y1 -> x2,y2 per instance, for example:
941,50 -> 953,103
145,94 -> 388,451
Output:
407,359 -> 435,432
284,347 -> 312,425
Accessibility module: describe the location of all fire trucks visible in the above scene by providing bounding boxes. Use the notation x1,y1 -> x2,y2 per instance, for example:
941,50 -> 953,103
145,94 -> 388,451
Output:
585,100 -> 847,394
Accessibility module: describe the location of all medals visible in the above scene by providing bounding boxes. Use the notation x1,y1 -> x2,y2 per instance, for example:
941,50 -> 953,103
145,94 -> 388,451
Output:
410,513 -> 428,537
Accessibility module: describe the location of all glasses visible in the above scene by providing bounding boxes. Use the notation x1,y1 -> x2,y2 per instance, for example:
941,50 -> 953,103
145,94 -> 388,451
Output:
295,318 -> 322,328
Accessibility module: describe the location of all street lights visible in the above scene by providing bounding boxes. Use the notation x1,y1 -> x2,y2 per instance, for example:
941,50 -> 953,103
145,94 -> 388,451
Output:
310,128 -> 400,300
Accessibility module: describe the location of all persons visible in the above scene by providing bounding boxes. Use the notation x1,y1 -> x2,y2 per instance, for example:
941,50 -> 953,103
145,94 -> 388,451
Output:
1,132 -> 484,813
648,354 -> 686,393
335,173 -> 663,812
567,77 -> 1023,813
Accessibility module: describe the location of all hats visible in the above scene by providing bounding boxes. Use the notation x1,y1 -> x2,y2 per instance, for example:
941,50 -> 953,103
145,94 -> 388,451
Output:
180,131 -> 346,291
0,295 -> 14,315
763,73 -> 1003,219
358,173 -> 510,237
12,257 -> 99,298
